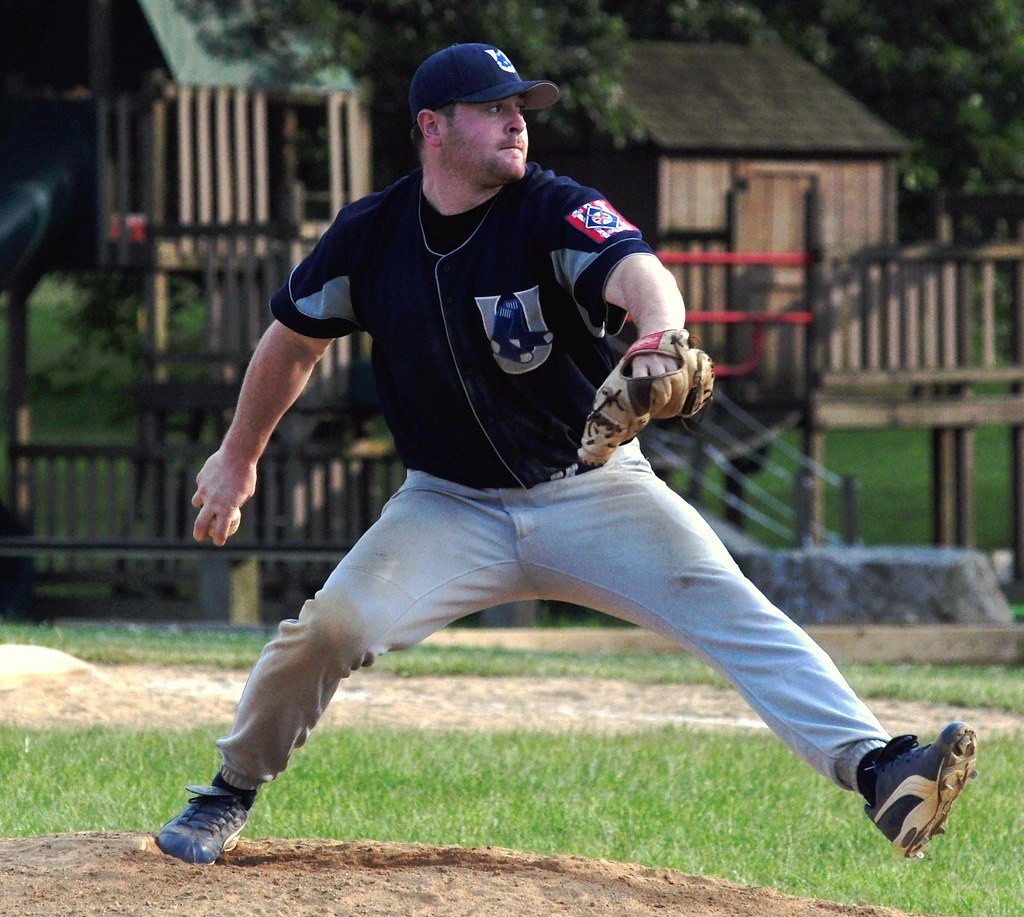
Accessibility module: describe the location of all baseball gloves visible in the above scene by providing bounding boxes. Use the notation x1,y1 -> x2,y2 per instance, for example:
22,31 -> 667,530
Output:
574,327 -> 717,470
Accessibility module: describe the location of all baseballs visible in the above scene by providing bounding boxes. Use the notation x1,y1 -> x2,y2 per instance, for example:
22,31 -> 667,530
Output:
206,507 -> 242,539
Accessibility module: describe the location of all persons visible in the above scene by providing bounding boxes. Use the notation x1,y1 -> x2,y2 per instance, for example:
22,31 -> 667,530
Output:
154,44 -> 977,863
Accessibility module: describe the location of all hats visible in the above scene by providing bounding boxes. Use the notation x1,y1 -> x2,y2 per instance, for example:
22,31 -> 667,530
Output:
408,42 -> 561,122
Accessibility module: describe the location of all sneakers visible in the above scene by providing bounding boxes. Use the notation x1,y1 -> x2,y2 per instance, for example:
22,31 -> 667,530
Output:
864,721 -> 979,858
155,785 -> 249,865
